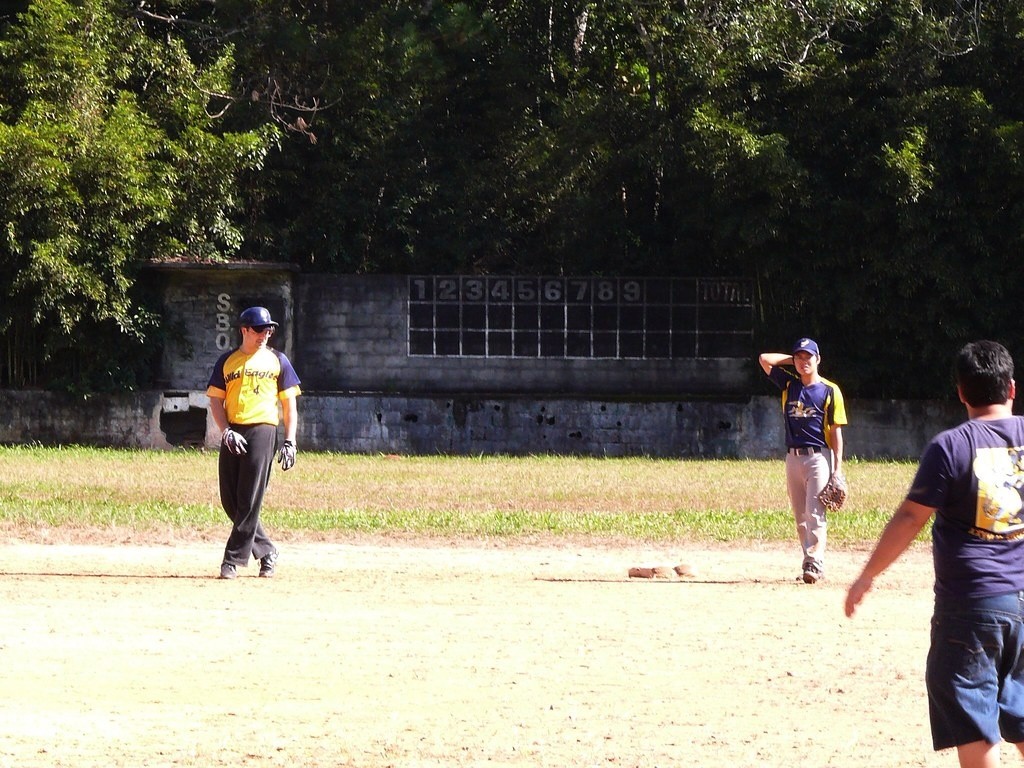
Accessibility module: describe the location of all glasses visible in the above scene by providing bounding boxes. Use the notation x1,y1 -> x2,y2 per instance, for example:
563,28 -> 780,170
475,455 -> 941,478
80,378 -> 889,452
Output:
251,325 -> 275,334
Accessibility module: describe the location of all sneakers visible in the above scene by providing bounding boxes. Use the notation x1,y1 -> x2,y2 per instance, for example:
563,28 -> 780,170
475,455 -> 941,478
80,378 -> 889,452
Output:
259,547 -> 279,577
220,562 -> 237,579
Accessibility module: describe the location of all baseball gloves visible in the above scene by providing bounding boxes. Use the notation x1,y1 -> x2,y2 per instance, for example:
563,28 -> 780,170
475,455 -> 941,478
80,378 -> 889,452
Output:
819,472 -> 848,514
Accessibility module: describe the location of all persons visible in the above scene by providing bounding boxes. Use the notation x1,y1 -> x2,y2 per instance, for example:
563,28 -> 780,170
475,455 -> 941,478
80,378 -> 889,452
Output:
759,338 -> 848,583
845,341 -> 1024,768
207,307 -> 302,579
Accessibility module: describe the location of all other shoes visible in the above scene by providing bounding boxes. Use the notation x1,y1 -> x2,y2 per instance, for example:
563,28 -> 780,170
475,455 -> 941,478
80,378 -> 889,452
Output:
801,563 -> 821,584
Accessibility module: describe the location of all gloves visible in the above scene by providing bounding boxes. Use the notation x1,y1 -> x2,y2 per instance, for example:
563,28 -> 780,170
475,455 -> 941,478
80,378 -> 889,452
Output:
221,427 -> 248,456
277,439 -> 296,471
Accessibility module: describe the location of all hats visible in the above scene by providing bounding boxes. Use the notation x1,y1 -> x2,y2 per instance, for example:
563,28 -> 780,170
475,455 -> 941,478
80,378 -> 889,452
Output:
791,338 -> 820,355
239,307 -> 279,326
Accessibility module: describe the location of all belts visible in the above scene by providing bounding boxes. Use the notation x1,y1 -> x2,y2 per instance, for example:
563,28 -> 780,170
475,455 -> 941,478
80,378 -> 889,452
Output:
787,446 -> 822,457
